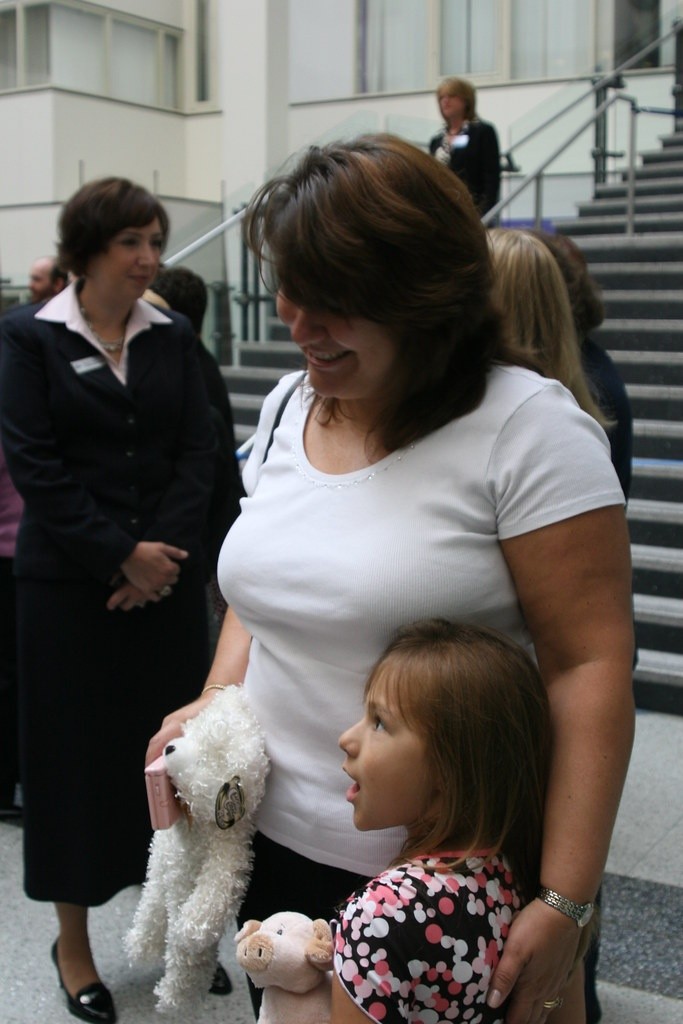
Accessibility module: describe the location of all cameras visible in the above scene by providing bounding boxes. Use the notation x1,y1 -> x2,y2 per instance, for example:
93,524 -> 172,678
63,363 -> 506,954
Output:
144,755 -> 183,830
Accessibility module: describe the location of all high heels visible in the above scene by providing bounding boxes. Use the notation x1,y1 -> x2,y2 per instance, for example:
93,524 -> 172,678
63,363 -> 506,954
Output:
208,962 -> 231,995
51,940 -> 115,1024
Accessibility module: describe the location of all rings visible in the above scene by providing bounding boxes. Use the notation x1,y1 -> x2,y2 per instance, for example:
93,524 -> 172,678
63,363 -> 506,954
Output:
160,586 -> 171,596
544,997 -> 559,1008
557,999 -> 564,1008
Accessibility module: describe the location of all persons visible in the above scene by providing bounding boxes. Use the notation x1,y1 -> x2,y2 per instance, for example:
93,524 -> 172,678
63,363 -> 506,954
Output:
0,78 -> 638,1024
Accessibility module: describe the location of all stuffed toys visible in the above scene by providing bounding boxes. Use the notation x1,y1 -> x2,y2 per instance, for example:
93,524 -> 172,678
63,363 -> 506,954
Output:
234,911 -> 342,1024
122,683 -> 272,1014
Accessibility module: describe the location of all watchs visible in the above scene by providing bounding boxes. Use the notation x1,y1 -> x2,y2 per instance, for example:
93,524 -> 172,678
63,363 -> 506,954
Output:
537,887 -> 594,927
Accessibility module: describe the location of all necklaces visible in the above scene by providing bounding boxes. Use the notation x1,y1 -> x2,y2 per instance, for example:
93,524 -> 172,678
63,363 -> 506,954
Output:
80,303 -> 127,351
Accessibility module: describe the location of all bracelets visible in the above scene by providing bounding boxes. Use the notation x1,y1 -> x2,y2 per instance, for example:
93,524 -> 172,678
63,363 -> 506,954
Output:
202,685 -> 226,696
